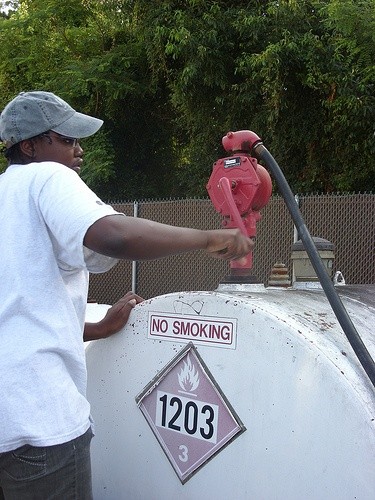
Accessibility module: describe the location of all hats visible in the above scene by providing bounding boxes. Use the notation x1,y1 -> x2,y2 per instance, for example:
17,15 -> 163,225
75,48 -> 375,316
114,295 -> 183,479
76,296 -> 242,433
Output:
0,90 -> 103,148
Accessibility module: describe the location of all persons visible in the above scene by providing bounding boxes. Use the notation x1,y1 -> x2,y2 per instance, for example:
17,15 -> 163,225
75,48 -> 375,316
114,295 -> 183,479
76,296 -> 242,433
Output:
0,91 -> 255,500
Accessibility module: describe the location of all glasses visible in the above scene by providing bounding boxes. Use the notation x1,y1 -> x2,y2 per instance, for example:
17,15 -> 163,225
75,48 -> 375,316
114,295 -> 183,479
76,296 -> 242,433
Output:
41,132 -> 81,148
